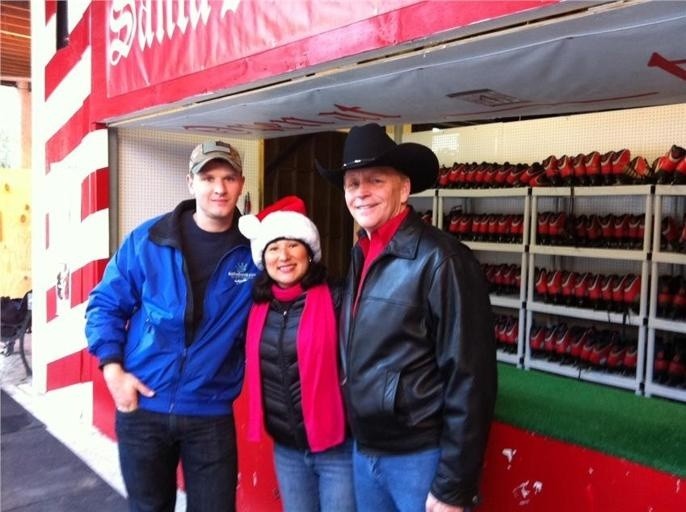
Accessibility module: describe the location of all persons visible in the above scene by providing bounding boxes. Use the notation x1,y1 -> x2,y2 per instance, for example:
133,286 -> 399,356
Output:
314,124 -> 497,511
85,140 -> 259,512
239,195 -> 354,511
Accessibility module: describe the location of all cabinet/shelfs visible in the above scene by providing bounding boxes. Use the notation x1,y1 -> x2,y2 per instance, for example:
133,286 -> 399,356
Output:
524,182 -> 654,397
436,188 -> 532,372
645,183 -> 686,404
408,188 -> 437,223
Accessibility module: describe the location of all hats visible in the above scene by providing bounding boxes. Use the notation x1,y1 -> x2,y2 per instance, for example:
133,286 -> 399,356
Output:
188,140 -> 242,175
314,123 -> 439,194
238,196 -> 322,272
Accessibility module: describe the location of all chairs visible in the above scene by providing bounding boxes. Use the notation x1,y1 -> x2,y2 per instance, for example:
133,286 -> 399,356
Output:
0,289 -> 31,388
